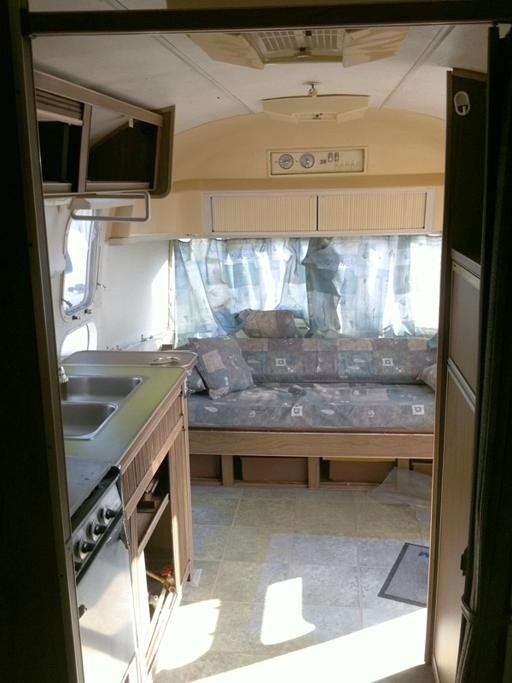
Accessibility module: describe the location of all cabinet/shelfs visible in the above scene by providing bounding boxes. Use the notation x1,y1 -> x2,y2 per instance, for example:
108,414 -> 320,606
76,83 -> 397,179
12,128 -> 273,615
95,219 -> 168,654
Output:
116,371 -> 194,682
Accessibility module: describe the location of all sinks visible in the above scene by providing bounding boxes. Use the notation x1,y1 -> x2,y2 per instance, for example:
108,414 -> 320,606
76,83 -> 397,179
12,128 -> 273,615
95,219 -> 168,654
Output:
61,403 -> 117,441
59,373 -> 149,400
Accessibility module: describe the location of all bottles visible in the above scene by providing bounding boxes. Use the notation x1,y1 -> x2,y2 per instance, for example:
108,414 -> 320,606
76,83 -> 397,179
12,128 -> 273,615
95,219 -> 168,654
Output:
58,365 -> 69,402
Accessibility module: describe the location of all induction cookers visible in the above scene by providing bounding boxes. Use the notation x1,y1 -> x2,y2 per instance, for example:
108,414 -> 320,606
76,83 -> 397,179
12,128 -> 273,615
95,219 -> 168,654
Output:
64,455 -> 122,577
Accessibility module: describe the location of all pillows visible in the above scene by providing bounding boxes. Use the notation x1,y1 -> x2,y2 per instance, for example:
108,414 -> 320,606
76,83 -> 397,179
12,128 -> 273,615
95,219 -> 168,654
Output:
234,308 -> 305,338
173,344 -> 207,394
416,360 -> 438,393
188,335 -> 257,401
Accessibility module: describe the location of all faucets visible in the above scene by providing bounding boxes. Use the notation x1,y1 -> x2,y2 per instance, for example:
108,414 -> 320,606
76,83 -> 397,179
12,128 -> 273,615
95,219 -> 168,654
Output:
58,366 -> 68,384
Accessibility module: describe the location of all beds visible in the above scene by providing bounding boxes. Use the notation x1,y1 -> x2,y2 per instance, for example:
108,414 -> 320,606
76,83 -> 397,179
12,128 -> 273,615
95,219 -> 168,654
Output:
173,337 -> 438,488
